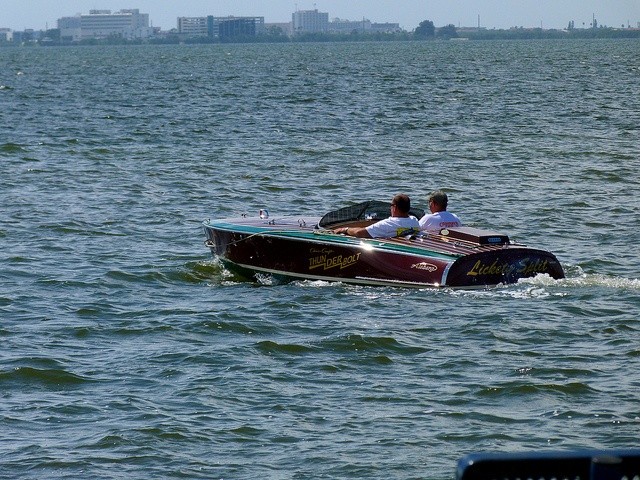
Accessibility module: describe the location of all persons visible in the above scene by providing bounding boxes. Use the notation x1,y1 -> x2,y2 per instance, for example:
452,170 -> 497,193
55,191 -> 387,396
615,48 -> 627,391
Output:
418,190 -> 462,230
332,194 -> 420,239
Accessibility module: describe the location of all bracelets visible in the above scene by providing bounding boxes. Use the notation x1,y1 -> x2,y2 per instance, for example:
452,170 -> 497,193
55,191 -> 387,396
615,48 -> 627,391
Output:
345,227 -> 348,235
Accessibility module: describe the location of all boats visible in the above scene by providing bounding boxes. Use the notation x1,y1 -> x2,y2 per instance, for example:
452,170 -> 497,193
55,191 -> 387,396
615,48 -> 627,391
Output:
202,199 -> 565,289
